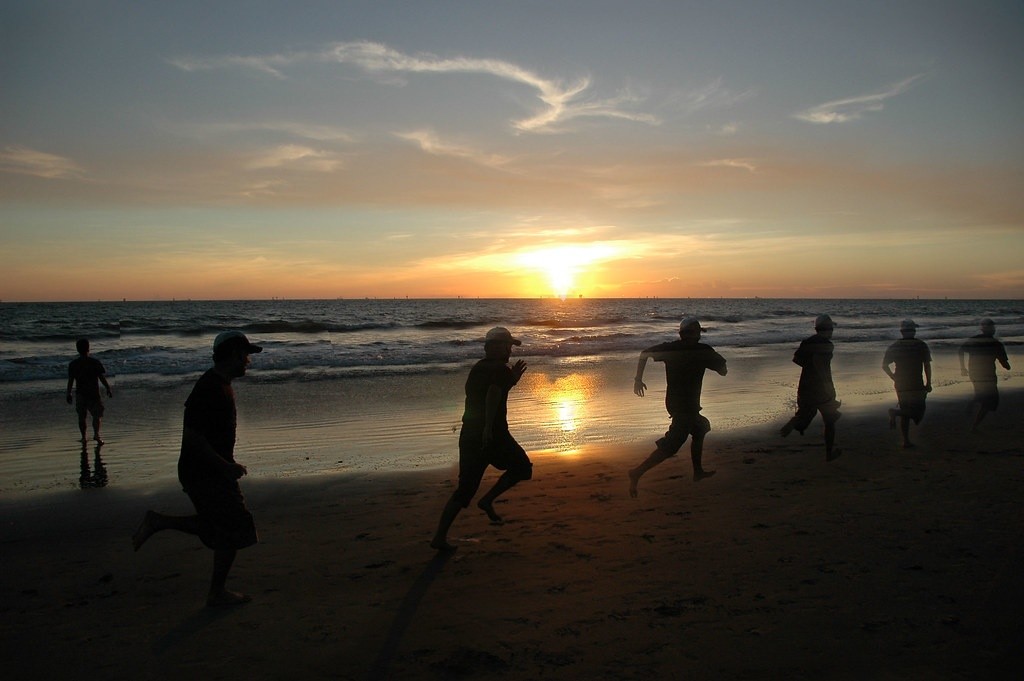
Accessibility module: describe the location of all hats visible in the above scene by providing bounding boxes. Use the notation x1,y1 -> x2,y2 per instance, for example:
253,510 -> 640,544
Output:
814,314 -> 837,327
486,327 -> 521,346
214,332 -> 263,354
900,319 -> 919,330
680,317 -> 707,333
979,318 -> 996,330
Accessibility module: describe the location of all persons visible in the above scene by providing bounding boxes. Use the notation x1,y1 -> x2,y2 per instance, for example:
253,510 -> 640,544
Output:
780,315 -> 842,462
67,338 -> 113,445
883,321 -> 932,448
131,330 -> 262,606
430,328 -> 532,550
959,319 -> 1010,432
629,317 -> 728,498
